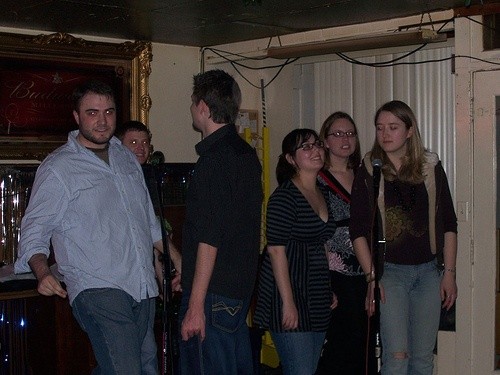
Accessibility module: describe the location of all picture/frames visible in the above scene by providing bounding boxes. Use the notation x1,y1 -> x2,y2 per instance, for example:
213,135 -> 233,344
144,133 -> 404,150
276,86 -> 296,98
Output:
0,31 -> 154,163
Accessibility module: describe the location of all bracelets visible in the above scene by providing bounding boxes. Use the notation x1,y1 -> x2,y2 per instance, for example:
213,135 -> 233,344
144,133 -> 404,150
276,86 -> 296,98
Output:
443,267 -> 455,272
364,272 -> 376,282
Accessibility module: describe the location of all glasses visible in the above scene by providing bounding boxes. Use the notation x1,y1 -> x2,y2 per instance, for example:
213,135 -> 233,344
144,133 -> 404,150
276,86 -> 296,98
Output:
295,141 -> 324,151
327,131 -> 356,137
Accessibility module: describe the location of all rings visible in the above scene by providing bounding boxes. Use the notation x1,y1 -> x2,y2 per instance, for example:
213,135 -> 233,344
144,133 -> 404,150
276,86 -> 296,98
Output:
446,295 -> 450,298
371,299 -> 375,303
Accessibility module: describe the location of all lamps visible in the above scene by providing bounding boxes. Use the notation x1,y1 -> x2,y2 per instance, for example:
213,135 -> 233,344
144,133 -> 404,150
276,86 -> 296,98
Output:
260,0 -> 448,60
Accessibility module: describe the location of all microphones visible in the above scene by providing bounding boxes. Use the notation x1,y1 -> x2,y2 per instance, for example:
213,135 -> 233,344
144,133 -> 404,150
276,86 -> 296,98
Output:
152,150 -> 173,182
370,159 -> 383,198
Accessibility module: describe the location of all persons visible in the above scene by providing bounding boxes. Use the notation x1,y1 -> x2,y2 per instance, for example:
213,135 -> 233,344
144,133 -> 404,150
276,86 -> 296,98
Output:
253,129 -> 338,375
172,70 -> 264,375
315,111 -> 377,375
349,100 -> 457,375
14,81 -> 182,375
115,121 -> 174,300
